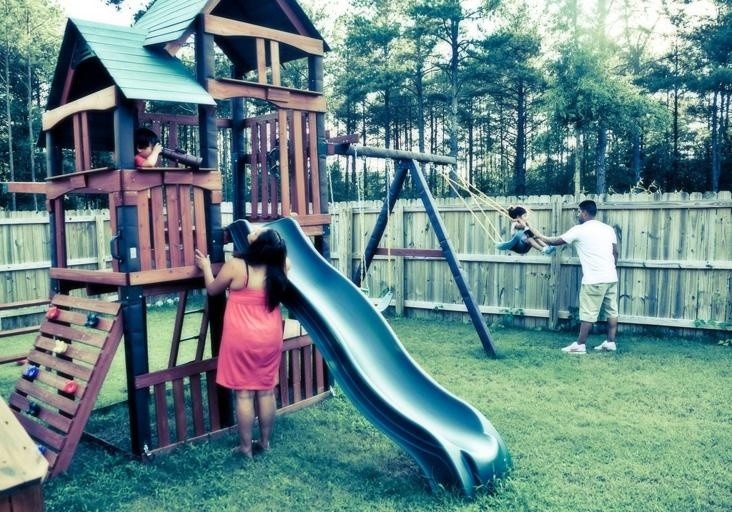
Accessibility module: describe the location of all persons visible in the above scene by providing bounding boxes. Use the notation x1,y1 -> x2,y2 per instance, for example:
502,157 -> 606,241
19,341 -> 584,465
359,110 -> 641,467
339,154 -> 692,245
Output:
135,129 -> 163,167
526,201 -> 618,354
508,207 -> 556,254
195,227 -> 292,457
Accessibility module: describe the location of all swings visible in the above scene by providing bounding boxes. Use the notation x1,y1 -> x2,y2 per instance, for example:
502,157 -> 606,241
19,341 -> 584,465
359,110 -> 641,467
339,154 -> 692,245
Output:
354,155 -> 393,314
429,164 -> 536,255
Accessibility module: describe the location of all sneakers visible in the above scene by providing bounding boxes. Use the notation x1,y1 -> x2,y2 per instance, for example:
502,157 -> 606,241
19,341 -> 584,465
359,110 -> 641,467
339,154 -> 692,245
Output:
561,342 -> 586,354
544,246 -> 557,254
592,341 -> 617,352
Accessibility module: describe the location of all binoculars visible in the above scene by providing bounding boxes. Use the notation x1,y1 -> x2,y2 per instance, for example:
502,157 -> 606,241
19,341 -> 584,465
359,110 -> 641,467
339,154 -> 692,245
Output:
160,147 -> 205,170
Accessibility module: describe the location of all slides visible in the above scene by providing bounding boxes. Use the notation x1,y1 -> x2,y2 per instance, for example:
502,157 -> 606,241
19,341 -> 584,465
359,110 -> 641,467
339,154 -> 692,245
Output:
223,217 -> 514,503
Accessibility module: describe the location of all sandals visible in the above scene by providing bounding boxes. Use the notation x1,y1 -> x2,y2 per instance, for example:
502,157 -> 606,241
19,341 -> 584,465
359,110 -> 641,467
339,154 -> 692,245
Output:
232,440 -> 269,459
